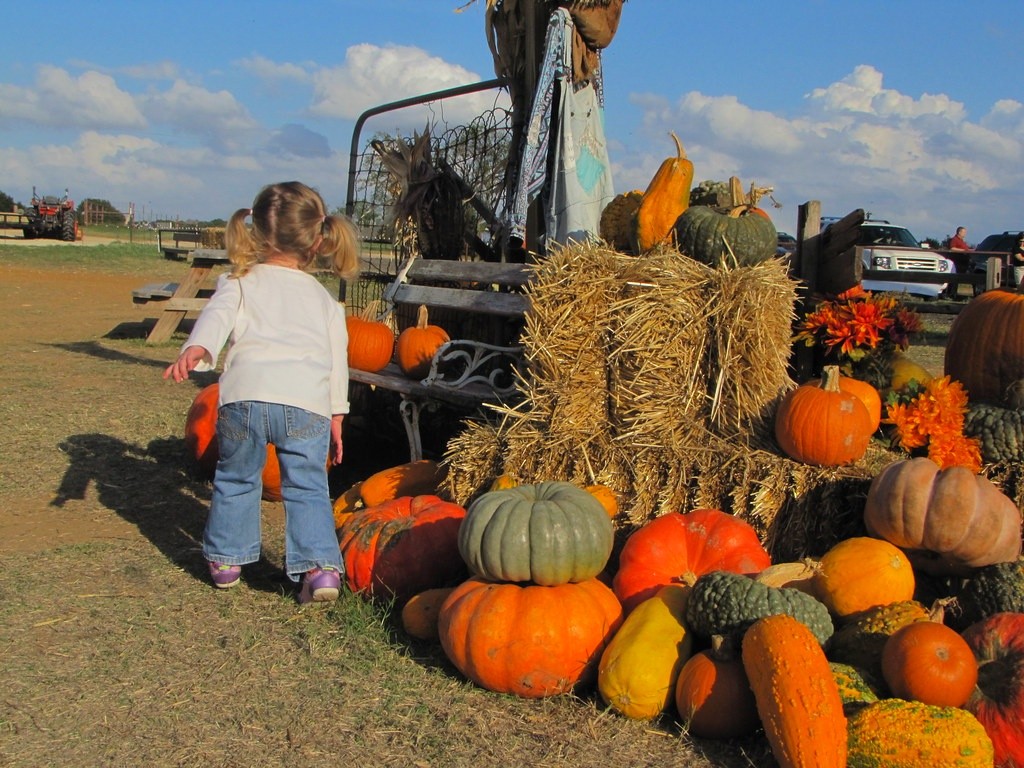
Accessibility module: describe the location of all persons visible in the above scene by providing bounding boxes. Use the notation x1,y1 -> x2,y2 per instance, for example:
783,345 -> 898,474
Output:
162,181 -> 359,609
1013,237 -> 1024,287
949,227 -> 970,273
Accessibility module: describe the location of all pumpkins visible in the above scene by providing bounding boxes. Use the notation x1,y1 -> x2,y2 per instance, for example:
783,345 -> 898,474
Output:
186,130 -> 1024,768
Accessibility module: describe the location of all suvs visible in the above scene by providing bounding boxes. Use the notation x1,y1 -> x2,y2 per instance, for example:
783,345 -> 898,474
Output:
966,231 -> 1020,296
778,232 -> 798,250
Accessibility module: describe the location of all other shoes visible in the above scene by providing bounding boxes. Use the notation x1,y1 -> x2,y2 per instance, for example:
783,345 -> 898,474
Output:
945,295 -> 955,300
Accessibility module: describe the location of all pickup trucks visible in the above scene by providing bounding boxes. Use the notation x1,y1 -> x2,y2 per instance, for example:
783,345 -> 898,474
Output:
819,218 -> 959,301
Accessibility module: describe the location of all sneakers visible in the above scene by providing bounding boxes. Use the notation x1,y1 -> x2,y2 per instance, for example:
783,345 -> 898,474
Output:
300,566 -> 340,608
208,561 -> 242,588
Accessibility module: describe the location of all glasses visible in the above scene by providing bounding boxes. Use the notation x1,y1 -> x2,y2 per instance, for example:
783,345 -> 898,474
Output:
1020,240 -> 1024,243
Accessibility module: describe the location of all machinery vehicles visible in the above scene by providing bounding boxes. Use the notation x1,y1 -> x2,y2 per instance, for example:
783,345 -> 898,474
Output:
23,196 -> 77,242
